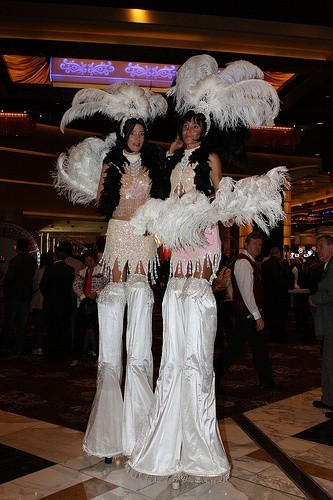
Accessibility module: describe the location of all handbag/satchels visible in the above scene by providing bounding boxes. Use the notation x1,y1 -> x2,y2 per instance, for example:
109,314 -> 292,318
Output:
79,297 -> 98,315
214,288 -> 227,303
236,306 -> 274,337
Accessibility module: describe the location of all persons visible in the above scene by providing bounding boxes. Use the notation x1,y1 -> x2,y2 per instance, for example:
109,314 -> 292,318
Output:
0,107 -> 333,488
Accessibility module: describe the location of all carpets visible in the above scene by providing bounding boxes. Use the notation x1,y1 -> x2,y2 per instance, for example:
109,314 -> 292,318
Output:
0,309 -> 322,432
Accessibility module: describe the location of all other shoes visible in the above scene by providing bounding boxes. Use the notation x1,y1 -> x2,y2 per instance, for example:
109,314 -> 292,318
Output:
313,400 -> 333,410
324,411 -> 333,420
87,349 -> 96,358
32,347 -> 43,356
258,381 -> 288,394
